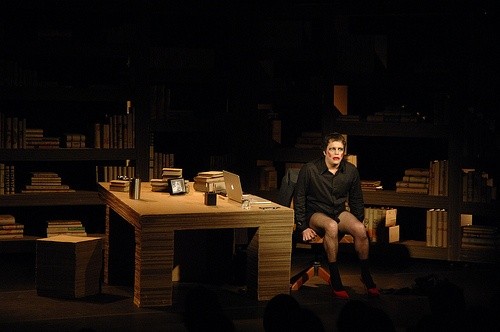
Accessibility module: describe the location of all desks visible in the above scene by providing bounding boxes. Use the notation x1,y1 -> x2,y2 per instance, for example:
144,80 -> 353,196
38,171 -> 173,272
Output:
99,182 -> 294,308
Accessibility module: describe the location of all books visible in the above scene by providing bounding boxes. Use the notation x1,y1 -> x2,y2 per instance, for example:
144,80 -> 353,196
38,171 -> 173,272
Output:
462,172 -> 496,203
0,114 -> 60,148
337,114 -> 418,123
346,206 -> 398,241
21,172 -> 75,192
256,103 -> 322,192
0,215 -> 24,239
193,171 -> 225,192
93,107 -> 135,148
461,226 -> 500,250
96,166 -> 140,200
43,220 -> 87,237
0,164 -> 15,194
345,155 -> 448,196
426,209 -> 448,247
63,133 -> 86,147
148,145 -> 190,192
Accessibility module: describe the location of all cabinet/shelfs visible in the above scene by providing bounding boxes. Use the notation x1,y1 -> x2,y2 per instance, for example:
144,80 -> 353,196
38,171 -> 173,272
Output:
0,48 -> 500,265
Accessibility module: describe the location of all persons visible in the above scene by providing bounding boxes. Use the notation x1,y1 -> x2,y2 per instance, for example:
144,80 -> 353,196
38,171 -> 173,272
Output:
184,279 -> 500,332
174,182 -> 182,192
292,133 -> 379,300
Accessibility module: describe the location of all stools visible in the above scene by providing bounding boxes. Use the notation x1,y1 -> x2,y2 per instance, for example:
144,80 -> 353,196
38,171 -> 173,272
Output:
36,233 -> 104,298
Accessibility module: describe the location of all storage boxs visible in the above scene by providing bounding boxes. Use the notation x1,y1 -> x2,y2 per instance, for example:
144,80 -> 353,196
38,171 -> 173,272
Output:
367,225 -> 400,243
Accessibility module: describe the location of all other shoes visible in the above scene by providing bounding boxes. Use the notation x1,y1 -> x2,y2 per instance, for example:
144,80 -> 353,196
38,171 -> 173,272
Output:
331,272 -> 342,290
362,270 -> 376,288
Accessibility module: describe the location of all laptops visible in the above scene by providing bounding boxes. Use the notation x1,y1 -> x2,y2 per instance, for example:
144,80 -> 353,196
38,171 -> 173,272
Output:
221,170 -> 271,205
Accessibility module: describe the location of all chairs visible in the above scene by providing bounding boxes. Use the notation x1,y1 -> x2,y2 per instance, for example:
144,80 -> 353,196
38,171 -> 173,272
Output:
290,197 -> 355,292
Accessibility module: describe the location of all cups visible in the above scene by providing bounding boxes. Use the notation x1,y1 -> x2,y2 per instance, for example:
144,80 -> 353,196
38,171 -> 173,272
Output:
204,192 -> 217,205
129,176 -> 141,200
